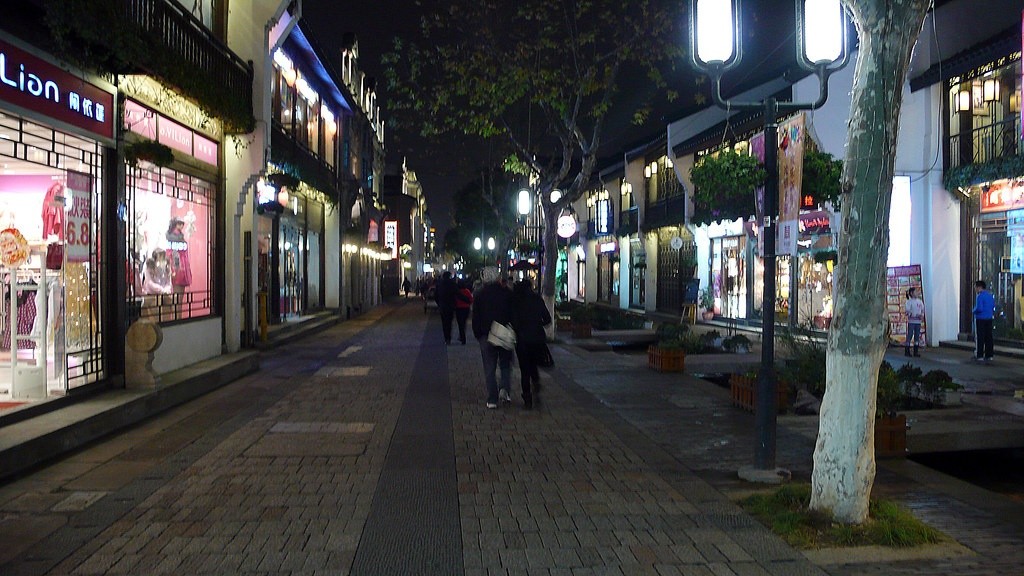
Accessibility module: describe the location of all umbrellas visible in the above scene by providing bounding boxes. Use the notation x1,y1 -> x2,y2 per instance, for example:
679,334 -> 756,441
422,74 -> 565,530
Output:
509,260 -> 538,271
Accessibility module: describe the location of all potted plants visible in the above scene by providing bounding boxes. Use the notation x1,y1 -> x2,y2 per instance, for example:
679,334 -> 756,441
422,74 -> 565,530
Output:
646,341 -> 686,374
268,172 -> 299,193
124,138 -> 175,167
873,367 -> 910,460
729,362 -> 789,416
554,302 -> 591,338
698,286 -> 715,321
256,200 -> 285,217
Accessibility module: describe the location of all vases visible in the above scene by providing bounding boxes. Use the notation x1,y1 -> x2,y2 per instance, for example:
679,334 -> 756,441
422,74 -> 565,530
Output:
720,193 -> 755,211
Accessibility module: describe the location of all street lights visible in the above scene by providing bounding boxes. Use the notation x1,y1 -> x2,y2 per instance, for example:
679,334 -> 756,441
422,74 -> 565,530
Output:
516,187 -> 563,295
474,236 -> 496,267
691,1 -> 850,473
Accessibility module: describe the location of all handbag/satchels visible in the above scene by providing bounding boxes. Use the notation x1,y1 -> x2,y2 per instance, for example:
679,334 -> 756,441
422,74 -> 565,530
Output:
487,321 -> 517,349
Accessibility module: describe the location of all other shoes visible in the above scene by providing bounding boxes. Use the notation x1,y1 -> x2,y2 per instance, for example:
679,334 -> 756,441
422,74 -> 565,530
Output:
531,384 -> 542,393
985,356 -> 993,361
486,401 -> 497,409
523,394 -> 533,409
976,357 -> 984,361
456,337 -> 466,345
499,389 -> 511,402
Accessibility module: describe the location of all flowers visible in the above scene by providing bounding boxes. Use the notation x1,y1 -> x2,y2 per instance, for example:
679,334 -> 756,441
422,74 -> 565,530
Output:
686,147 -> 769,218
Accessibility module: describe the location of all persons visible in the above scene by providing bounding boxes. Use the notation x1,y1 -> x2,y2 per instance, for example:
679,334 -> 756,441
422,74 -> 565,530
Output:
414,276 -> 425,296
472,277 -> 553,411
434,271 -> 473,345
402,277 -> 411,298
971,280 -> 996,363
904,288 -> 924,357
142,248 -> 172,322
166,217 -> 192,320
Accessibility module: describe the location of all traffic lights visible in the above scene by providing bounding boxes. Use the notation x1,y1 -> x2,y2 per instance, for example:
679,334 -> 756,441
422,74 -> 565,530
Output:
800,191 -> 819,210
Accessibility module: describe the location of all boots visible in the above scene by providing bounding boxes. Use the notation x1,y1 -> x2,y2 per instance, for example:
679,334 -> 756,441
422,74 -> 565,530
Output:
905,346 -> 913,358
914,345 -> 921,357
